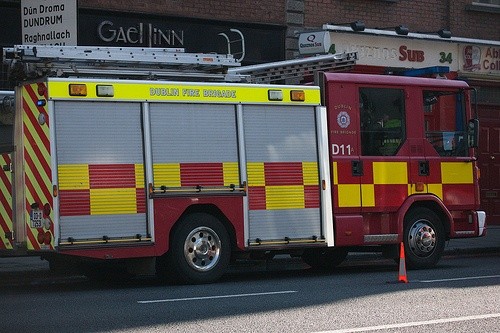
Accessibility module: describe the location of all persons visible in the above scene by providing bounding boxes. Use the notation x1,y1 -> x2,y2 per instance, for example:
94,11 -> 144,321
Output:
359,102 -> 390,132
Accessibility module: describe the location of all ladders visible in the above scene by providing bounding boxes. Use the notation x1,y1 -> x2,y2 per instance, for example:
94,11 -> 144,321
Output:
1,42 -> 254,82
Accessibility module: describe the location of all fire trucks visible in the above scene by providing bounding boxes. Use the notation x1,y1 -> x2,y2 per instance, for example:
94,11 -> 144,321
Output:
0,29 -> 485,281
